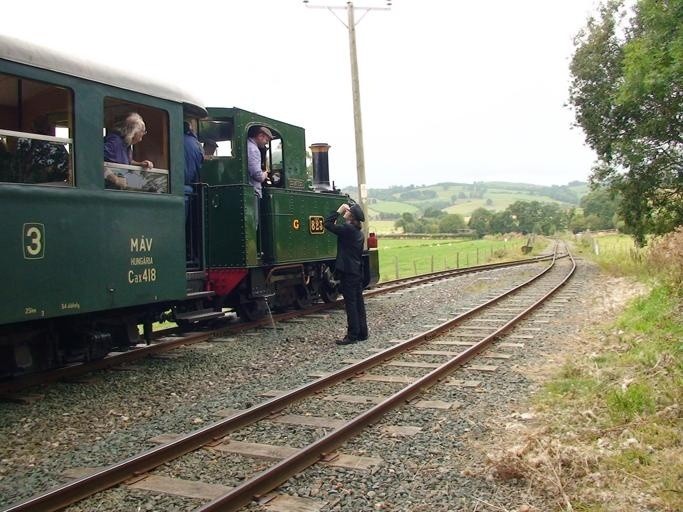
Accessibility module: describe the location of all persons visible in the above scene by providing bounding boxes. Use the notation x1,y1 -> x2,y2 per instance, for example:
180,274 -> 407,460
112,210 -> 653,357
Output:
183,120 -> 205,221
103,112 -> 153,189
323,202 -> 368,345
247,126 -> 274,231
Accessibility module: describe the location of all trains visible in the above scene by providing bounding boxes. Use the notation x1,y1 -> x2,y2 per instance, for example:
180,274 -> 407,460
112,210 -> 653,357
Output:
0,36 -> 382,385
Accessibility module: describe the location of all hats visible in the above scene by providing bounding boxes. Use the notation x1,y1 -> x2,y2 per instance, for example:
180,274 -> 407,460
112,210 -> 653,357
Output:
260,127 -> 273,140
351,203 -> 366,222
204,139 -> 218,147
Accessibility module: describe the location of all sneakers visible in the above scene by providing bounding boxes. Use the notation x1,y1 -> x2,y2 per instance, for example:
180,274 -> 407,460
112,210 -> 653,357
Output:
335,335 -> 368,345
